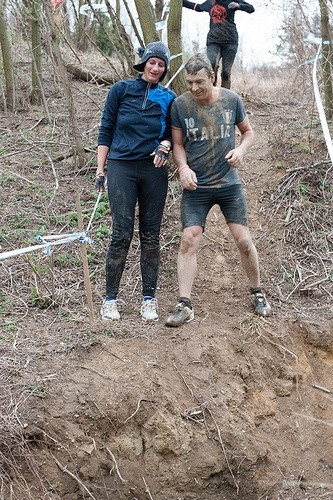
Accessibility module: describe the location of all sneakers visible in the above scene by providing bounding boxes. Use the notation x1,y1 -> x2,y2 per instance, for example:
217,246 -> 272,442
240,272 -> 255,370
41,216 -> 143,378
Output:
100,298 -> 125,322
140,297 -> 160,322
164,302 -> 195,327
250,287 -> 273,317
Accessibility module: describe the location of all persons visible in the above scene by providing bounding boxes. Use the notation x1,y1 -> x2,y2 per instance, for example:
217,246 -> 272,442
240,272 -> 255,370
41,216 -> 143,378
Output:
165,54 -> 271,326
95,42 -> 177,321
182,0 -> 255,90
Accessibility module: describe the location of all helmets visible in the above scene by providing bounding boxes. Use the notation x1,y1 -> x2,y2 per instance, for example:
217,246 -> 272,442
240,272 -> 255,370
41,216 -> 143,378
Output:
132,41 -> 171,83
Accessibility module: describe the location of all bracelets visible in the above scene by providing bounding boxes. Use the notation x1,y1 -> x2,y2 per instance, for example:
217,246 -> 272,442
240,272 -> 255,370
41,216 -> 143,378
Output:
178,165 -> 189,173
